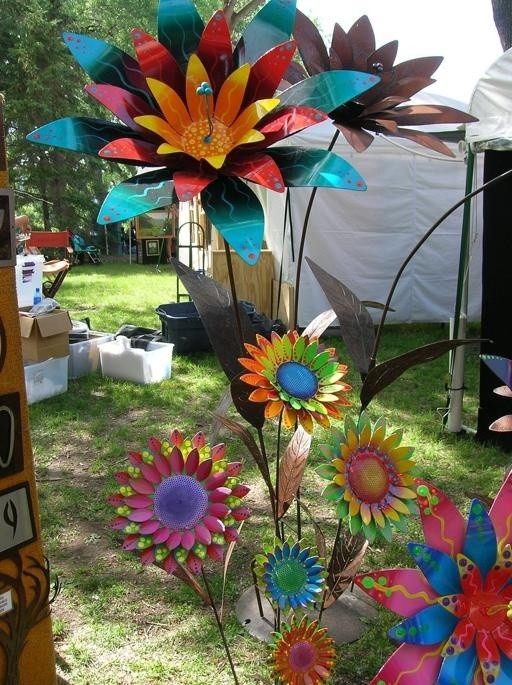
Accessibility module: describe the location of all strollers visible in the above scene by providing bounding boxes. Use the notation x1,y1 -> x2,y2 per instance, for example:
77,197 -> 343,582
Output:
66,227 -> 101,264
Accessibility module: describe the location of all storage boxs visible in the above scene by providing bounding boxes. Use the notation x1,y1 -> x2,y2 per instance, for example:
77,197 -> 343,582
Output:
14,253 -> 214,407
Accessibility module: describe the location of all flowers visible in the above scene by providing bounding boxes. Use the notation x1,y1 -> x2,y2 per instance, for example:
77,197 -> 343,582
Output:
26,1 -> 512,685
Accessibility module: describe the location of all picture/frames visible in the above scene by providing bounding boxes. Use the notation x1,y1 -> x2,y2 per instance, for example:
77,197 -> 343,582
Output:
0,187 -> 17,268
0,480 -> 38,561
135,209 -> 176,240
145,240 -> 160,257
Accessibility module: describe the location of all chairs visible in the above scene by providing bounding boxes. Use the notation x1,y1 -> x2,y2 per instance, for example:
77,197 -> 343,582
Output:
68,230 -> 103,264
23,226 -> 74,299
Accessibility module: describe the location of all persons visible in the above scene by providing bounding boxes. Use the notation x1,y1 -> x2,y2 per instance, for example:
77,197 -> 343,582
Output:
124,220 -> 137,256
163,202 -> 179,264
